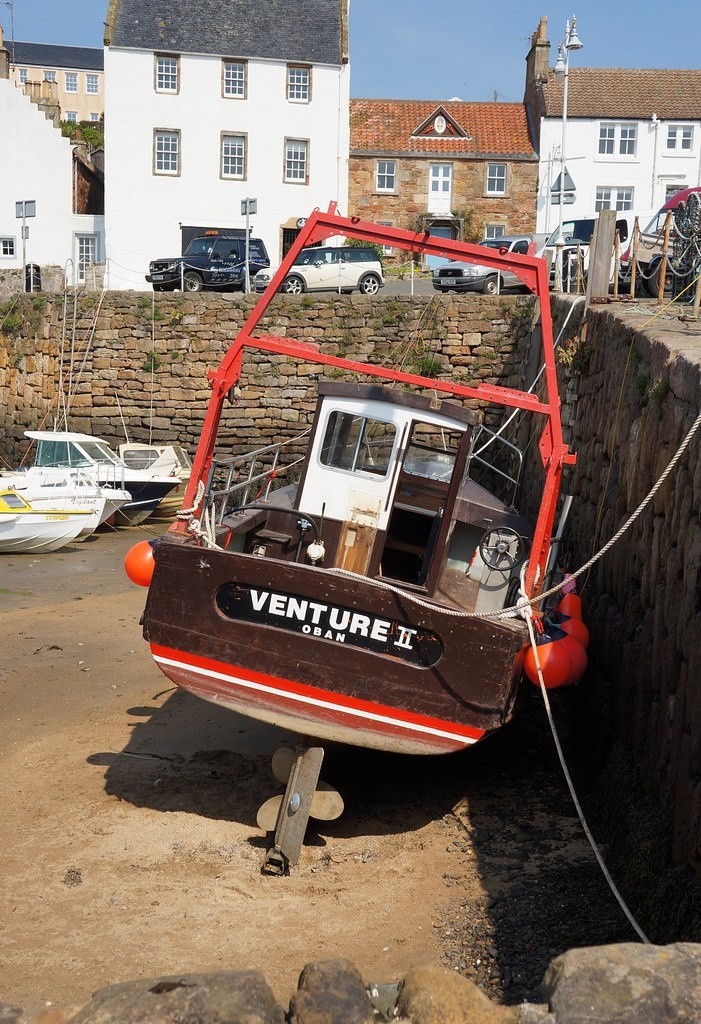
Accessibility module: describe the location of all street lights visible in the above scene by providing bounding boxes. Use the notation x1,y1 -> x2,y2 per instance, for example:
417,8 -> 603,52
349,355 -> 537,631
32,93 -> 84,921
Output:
552,10 -> 584,293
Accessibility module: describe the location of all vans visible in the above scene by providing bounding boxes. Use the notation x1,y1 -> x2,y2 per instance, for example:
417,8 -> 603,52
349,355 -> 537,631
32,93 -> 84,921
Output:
621,186 -> 701,299
533,208 -> 675,297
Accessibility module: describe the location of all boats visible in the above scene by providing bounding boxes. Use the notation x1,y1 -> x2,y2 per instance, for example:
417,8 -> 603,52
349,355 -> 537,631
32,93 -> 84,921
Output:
122,197 -> 592,881
0,272 -> 195,556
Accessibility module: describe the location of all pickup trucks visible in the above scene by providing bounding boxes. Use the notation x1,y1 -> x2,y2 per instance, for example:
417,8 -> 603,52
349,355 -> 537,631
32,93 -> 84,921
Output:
431,231 -> 576,296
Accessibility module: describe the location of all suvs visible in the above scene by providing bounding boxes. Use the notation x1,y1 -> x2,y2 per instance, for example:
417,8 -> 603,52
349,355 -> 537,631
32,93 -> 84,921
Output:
253,245 -> 387,296
145,230 -> 270,292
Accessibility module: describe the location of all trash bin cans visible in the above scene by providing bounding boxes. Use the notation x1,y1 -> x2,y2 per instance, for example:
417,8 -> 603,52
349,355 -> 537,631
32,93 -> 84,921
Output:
26,261 -> 42,292
